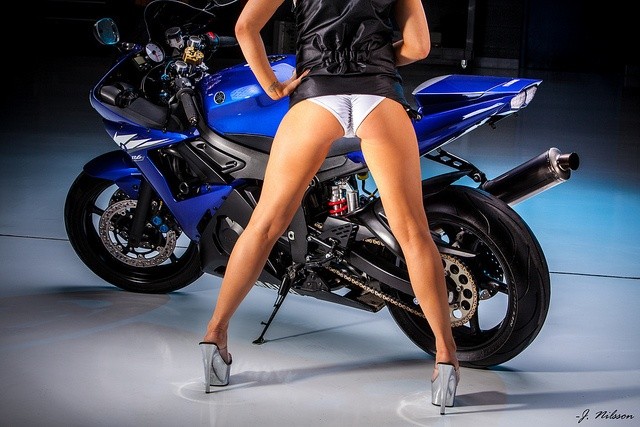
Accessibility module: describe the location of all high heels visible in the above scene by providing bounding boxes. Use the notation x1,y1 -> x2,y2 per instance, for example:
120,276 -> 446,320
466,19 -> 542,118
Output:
199,341 -> 232,393
431,364 -> 459,416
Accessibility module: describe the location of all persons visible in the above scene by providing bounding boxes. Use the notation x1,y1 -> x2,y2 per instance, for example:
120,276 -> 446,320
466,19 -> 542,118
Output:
200,0 -> 461,415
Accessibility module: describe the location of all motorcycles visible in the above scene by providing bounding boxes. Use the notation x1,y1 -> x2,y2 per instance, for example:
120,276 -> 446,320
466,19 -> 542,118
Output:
63,0 -> 580,369
